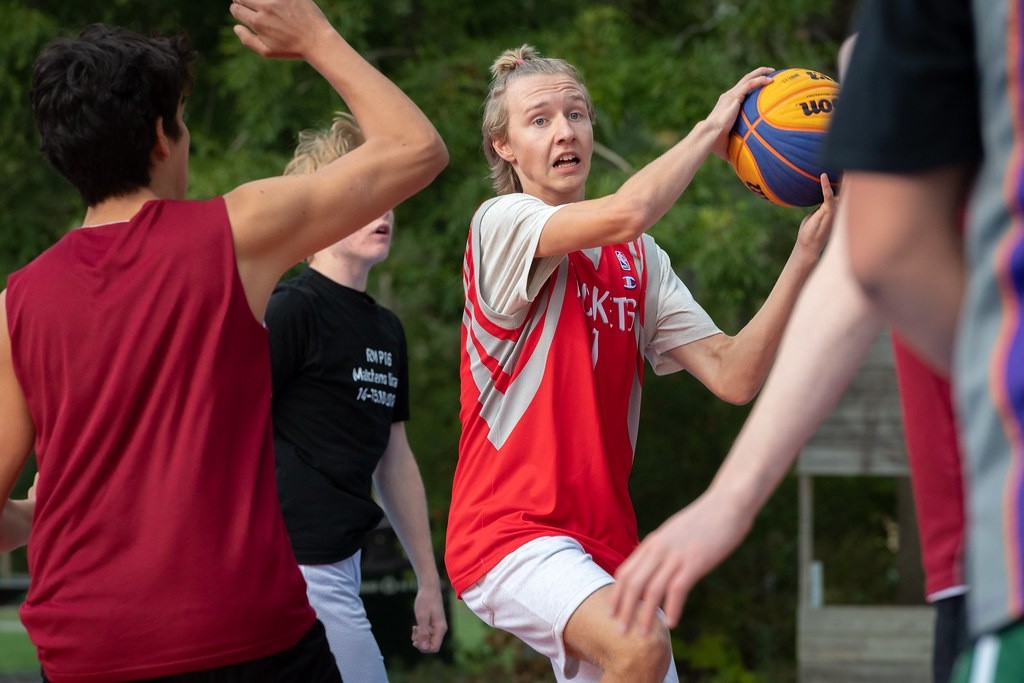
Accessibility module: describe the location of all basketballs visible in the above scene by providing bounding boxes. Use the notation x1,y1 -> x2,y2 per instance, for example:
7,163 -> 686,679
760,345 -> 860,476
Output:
727,67 -> 841,209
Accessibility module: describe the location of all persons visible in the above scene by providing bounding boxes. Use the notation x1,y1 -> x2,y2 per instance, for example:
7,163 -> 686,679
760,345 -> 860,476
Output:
442,43 -> 842,683
0,0 -> 448,683
608,0 -> 1024,683
